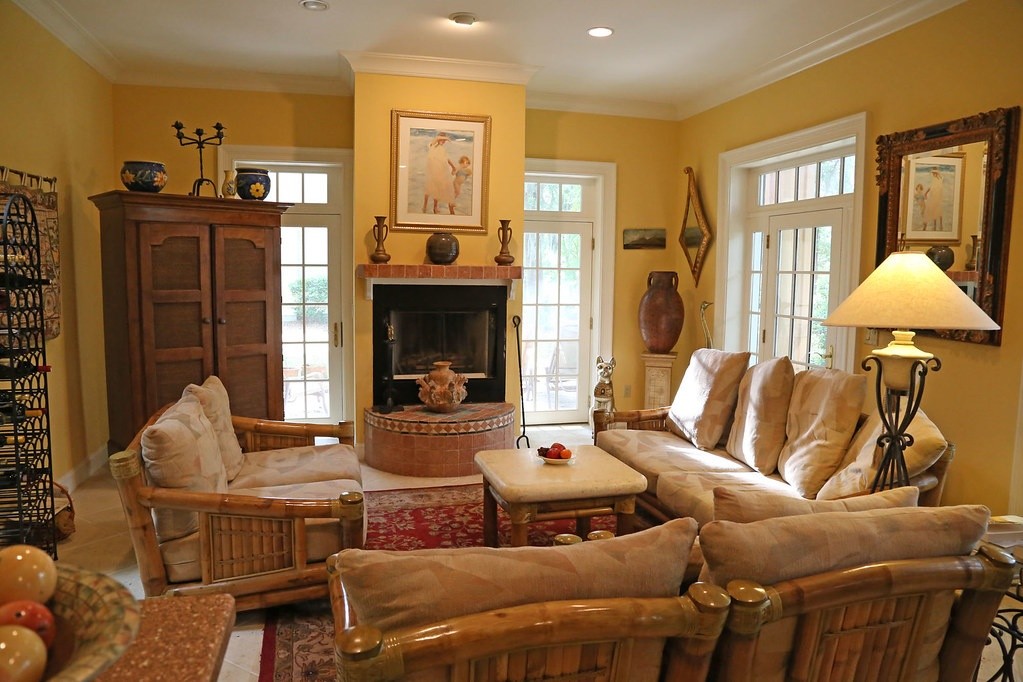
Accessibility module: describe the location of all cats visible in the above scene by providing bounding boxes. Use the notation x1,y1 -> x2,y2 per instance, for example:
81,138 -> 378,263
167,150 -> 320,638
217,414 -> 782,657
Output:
589,355 -> 617,439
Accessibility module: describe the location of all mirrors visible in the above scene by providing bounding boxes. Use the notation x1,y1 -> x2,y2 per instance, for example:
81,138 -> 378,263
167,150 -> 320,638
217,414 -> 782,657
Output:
678,167 -> 711,287
874,106 -> 1020,344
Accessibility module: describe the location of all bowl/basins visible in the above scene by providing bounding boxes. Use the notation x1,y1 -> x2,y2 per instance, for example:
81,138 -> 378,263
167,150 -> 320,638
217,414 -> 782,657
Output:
234,167 -> 271,201
121,160 -> 168,192
43,559 -> 141,681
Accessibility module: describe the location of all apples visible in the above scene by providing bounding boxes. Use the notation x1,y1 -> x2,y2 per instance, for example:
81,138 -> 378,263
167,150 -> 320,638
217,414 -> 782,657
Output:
546,443 -> 565,459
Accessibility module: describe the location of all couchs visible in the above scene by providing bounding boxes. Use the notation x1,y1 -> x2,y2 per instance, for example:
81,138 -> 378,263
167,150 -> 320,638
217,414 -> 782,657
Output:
112,381 -> 366,608
325,534 -> 730,682
592,502 -> 1014,682
590,402 -> 955,536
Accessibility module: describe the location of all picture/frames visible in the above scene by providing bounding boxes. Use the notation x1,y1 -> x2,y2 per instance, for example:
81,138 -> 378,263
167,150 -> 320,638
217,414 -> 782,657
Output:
898,150 -> 967,246
389,110 -> 493,234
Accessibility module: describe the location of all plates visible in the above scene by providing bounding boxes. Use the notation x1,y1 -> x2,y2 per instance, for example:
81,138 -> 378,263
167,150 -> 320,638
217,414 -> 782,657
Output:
535,452 -> 576,465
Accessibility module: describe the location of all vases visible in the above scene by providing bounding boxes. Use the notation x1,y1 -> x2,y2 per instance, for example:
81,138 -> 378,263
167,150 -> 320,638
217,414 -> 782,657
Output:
494,219 -> 514,266
417,361 -> 468,413
927,245 -> 955,271
426,233 -> 458,265
966,235 -> 979,270
222,170 -> 236,198
370,215 -> 391,263
235,167 -> 271,200
638,271 -> 684,354
123,160 -> 167,192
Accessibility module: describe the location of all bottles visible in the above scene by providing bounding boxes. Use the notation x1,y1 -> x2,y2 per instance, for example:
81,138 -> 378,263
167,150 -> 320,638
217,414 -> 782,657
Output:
637,272 -> 685,354
926,245 -> 954,271
222,170 -> 237,198
426,233 -> 459,265
966,235 -> 977,269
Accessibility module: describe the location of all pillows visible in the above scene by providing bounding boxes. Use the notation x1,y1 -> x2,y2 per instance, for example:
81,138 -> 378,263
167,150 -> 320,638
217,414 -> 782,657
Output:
665,347 -> 751,452
335,484 -> 989,632
815,396 -> 949,500
143,376 -> 243,542
777,367 -> 866,499
725,355 -> 795,474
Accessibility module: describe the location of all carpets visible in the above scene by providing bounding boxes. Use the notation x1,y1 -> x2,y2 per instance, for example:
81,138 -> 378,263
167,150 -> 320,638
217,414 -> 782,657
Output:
257,482 -> 616,682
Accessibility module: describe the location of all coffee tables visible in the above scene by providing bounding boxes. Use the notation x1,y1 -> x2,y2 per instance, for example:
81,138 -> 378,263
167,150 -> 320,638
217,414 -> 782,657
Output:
474,443 -> 648,548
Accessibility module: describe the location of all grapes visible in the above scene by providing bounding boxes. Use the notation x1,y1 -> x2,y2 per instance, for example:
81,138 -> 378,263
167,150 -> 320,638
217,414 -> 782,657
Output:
538,447 -> 550,456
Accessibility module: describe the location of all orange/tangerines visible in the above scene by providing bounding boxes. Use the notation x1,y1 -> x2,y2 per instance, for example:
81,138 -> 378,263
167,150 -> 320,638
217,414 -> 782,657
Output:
560,449 -> 571,459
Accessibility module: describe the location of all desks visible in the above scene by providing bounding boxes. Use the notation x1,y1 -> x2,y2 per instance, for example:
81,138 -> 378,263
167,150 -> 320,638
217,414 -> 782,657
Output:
89,594 -> 236,682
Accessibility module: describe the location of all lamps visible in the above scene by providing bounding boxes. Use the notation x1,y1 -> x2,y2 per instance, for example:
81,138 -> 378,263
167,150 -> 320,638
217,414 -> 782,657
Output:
820,251 -> 1001,494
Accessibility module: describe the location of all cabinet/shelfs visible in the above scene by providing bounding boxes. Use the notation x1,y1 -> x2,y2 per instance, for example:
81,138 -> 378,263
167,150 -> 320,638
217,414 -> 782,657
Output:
0,194 -> 60,559
88,189 -> 296,456
